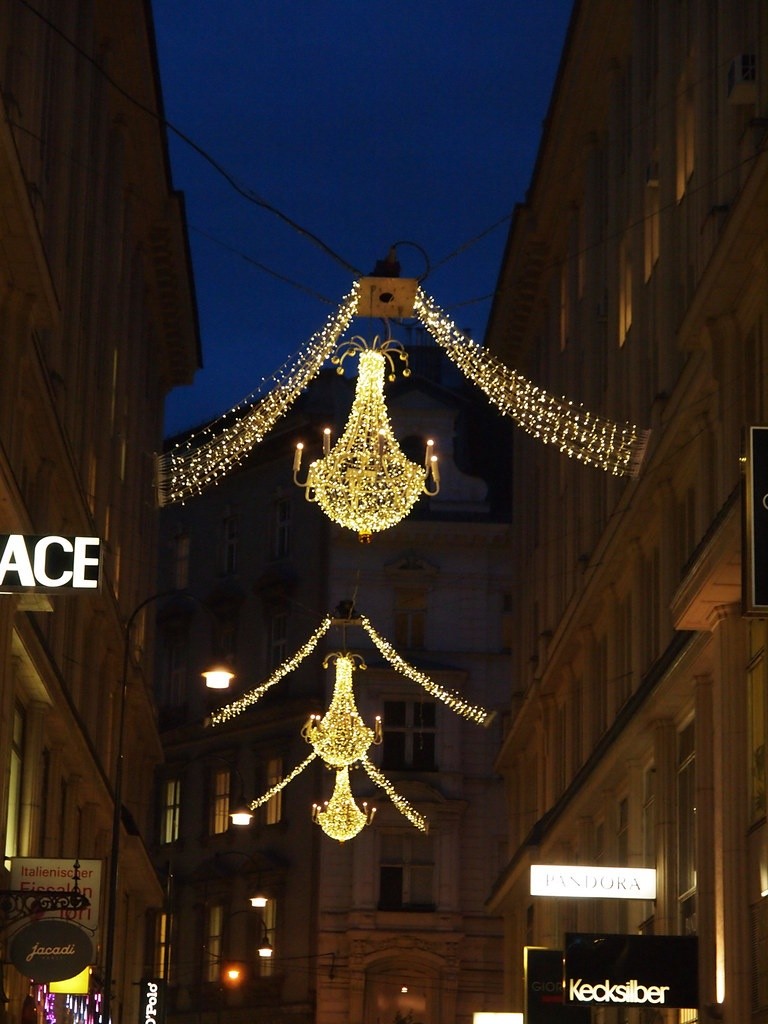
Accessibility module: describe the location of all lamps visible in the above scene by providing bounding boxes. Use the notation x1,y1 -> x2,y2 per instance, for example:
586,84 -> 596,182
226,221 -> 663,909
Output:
248,746 -> 429,842
204,601 -> 496,770
160,238 -> 642,547
